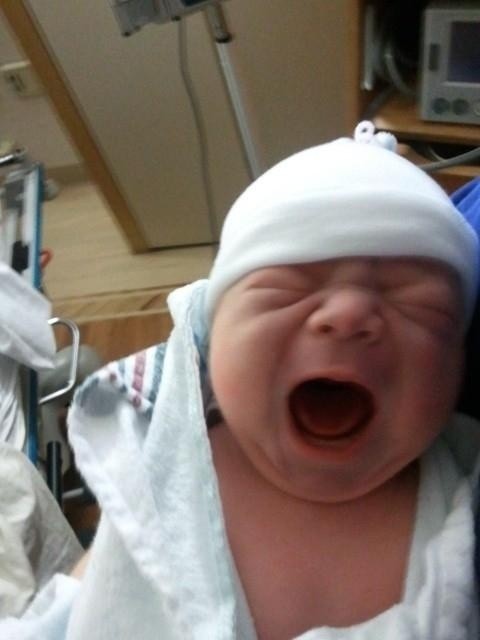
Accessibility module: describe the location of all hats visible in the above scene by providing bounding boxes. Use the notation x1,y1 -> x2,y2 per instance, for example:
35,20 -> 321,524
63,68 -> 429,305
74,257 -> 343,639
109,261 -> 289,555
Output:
202,121 -> 479,316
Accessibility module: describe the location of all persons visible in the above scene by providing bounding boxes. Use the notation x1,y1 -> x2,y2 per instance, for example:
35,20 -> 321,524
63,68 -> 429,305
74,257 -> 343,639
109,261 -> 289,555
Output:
63,118 -> 478,640
